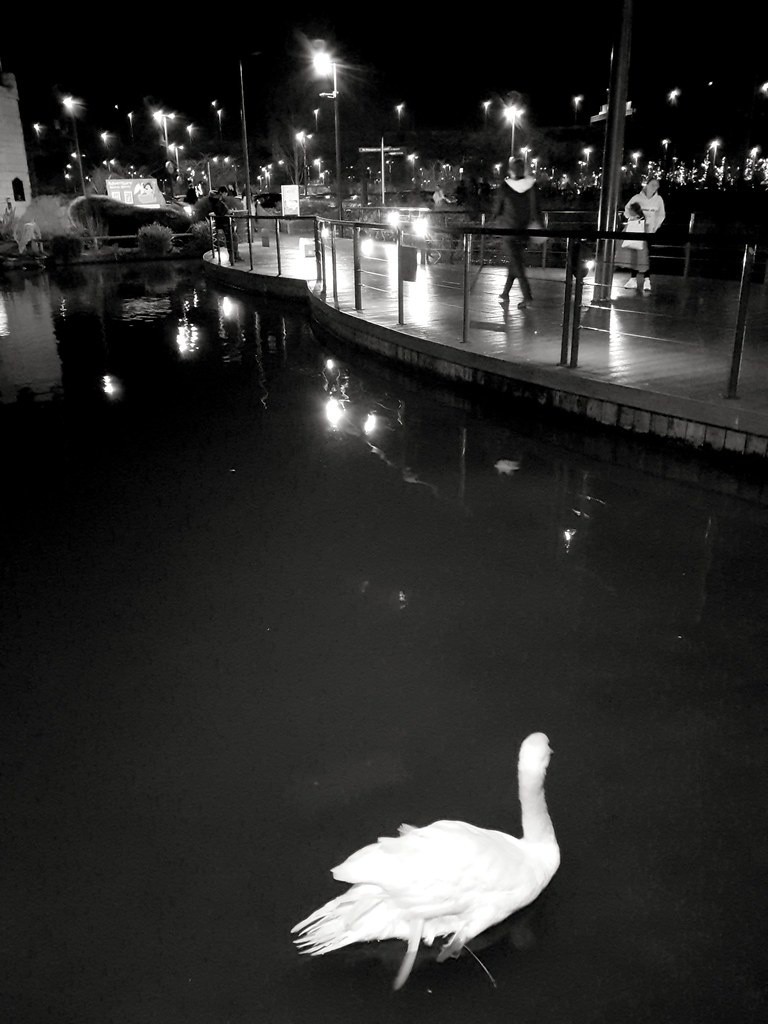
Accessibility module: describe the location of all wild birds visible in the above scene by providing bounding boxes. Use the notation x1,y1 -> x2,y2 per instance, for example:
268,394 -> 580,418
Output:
287,732 -> 562,990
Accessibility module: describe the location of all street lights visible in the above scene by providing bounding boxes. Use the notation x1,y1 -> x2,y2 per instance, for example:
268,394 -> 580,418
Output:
304,38 -> 343,235
500,99 -> 525,152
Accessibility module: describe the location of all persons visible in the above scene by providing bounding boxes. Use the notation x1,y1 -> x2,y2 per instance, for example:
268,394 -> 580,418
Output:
432,185 -> 457,208
623,178 -> 666,291
3,198 -> 16,224
478,177 -> 491,203
453,180 -> 468,206
183,180 -> 204,205
208,185 -> 245,262
488,159 -> 546,309
